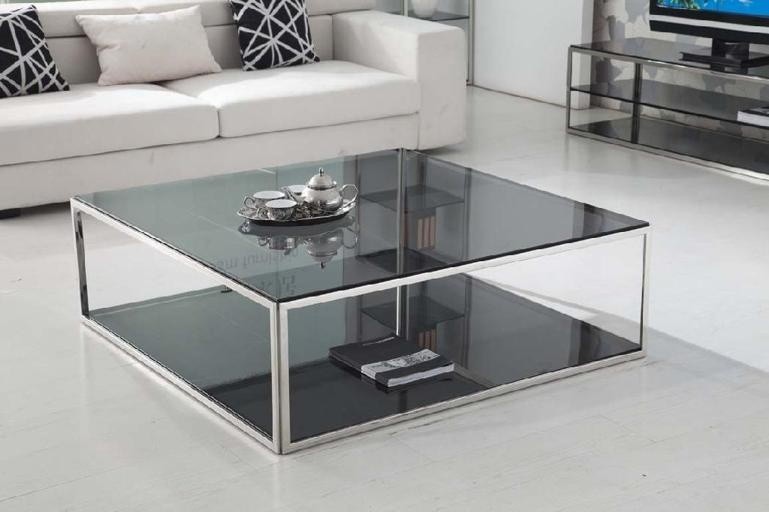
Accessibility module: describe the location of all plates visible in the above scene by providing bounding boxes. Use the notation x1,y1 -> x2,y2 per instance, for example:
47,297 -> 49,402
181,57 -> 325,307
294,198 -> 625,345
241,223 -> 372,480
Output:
239,213 -> 357,237
237,197 -> 354,227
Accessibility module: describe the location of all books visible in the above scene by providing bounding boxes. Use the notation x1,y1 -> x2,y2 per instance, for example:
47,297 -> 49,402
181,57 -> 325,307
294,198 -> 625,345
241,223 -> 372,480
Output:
732,104 -> 768,130
329,357 -> 457,393
328,331 -> 454,386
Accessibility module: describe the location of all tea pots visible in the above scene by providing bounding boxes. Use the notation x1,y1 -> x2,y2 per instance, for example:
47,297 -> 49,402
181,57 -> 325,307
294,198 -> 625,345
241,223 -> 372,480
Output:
300,227 -> 360,270
282,166 -> 358,215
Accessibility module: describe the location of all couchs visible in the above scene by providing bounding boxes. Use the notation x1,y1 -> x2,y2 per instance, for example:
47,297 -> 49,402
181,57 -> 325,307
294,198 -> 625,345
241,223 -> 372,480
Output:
0,0 -> 468,212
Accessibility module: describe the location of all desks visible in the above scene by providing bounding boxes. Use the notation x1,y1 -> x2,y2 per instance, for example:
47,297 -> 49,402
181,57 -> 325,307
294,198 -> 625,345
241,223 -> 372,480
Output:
387,8 -> 474,85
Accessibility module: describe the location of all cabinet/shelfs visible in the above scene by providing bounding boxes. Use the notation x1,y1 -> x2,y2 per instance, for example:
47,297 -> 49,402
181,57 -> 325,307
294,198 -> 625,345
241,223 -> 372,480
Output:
565,37 -> 769,182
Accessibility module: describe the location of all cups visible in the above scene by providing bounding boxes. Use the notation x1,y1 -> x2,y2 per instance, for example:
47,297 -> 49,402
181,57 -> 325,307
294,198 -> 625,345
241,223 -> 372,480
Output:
280,185 -> 306,196
243,190 -> 285,210
255,199 -> 300,222
257,236 -> 297,251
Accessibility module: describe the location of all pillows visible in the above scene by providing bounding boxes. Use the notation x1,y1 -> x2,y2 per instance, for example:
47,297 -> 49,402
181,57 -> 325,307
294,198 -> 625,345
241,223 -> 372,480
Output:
76,5 -> 222,86
226,0 -> 320,72
0,4 -> 69,99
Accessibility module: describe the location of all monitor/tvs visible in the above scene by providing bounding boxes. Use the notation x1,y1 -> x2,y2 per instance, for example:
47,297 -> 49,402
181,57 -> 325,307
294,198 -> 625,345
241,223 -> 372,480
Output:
650,0 -> 769,68
